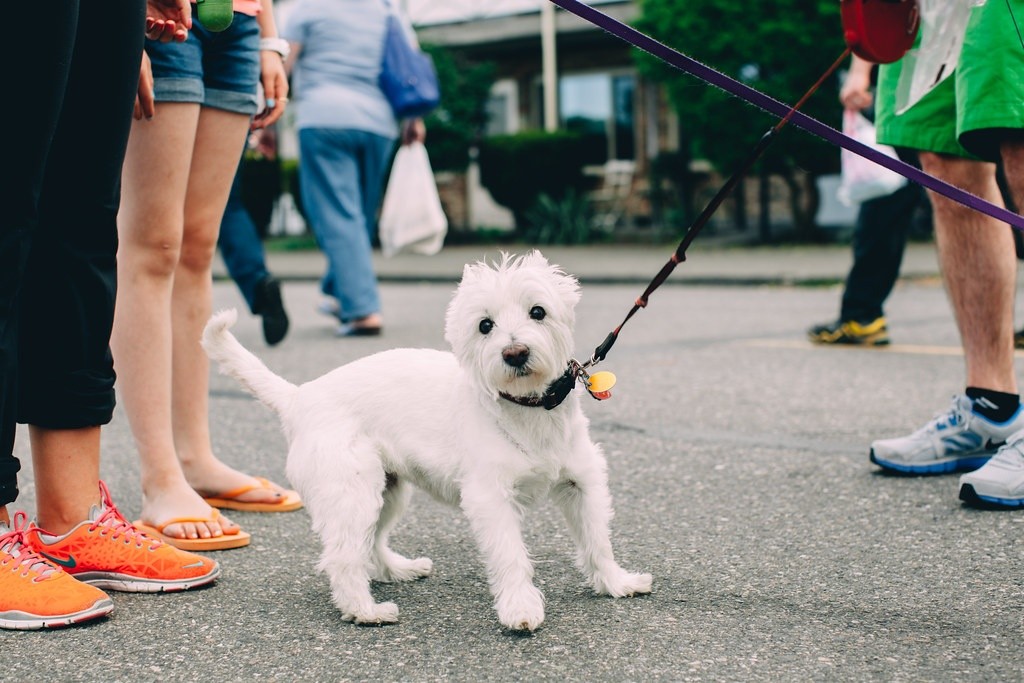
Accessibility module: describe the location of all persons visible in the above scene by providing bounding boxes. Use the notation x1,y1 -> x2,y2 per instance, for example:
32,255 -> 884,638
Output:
869,0 -> 1024,508
0,1 -> 226,630
216,121 -> 290,347
271,1 -> 429,337
108,1 -> 306,552
806,52 -> 1024,350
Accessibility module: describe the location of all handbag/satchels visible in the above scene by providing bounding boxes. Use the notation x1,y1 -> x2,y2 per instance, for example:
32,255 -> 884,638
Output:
378,139 -> 448,260
835,106 -> 909,209
379,0 -> 441,121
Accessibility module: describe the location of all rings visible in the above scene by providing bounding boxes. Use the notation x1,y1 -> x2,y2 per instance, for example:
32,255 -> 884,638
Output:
275,97 -> 289,104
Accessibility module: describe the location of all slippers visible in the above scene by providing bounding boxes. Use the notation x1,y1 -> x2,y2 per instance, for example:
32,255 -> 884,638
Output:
203,477 -> 303,512
334,321 -> 380,337
317,300 -> 349,323
133,507 -> 252,551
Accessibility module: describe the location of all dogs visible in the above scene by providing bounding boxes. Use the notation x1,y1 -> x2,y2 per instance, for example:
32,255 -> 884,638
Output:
202,248 -> 655,634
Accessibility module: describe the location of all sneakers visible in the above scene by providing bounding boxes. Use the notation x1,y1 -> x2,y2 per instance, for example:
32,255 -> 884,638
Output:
0,520 -> 115,631
806,316 -> 891,346
957,425 -> 1024,509
258,276 -> 289,346
1013,325 -> 1024,351
868,396 -> 1023,474
24,498 -> 221,592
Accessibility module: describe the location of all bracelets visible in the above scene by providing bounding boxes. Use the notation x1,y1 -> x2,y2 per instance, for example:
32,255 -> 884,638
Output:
259,37 -> 291,63
